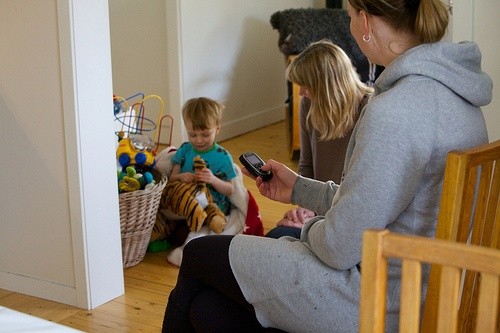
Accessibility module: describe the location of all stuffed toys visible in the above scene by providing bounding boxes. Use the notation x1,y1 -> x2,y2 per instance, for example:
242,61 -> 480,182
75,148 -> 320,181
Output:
150,154 -> 228,241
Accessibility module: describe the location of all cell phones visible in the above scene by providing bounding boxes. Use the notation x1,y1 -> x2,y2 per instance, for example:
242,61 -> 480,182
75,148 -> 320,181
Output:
239,152 -> 273,181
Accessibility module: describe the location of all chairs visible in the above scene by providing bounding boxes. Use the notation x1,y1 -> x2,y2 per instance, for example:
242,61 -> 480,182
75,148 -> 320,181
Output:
359,141 -> 500,333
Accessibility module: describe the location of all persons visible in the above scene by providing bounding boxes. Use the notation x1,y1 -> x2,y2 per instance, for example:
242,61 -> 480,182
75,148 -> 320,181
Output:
161,0 -> 493,333
265,40 -> 374,238
167,97 -> 237,249
